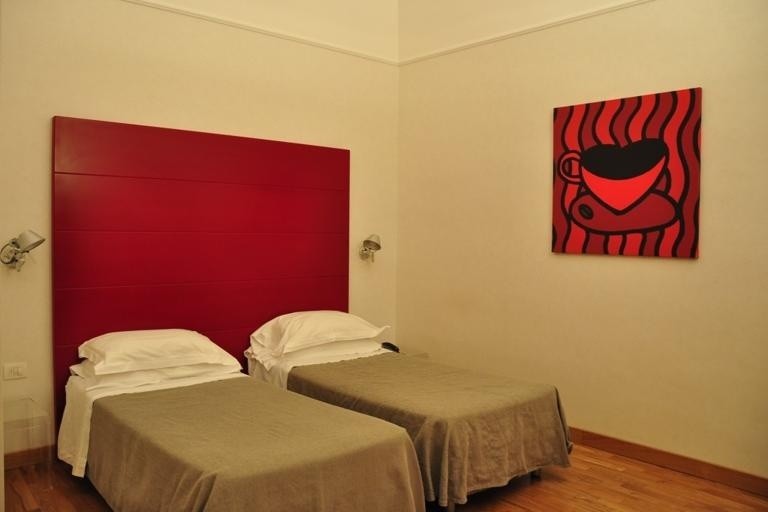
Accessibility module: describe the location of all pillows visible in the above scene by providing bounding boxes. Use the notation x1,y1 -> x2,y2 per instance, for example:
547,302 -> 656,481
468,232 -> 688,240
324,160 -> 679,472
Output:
68,329 -> 243,391
244,310 -> 392,374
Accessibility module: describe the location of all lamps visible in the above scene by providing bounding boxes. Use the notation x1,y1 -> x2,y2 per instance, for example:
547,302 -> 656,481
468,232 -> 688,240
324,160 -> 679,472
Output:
0,229 -> 45,269
361,235 -> 382,262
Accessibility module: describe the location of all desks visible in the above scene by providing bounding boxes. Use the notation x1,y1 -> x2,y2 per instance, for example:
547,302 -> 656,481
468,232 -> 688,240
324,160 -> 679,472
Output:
0,396 -> 53,494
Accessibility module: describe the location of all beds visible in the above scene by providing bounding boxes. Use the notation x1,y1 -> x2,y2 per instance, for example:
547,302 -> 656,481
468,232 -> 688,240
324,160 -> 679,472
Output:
245,351 -> 569,512
57,374 -> 424,512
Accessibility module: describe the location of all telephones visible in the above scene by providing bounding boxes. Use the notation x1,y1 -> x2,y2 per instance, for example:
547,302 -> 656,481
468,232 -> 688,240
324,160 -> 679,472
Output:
382,342 -> 399,353
